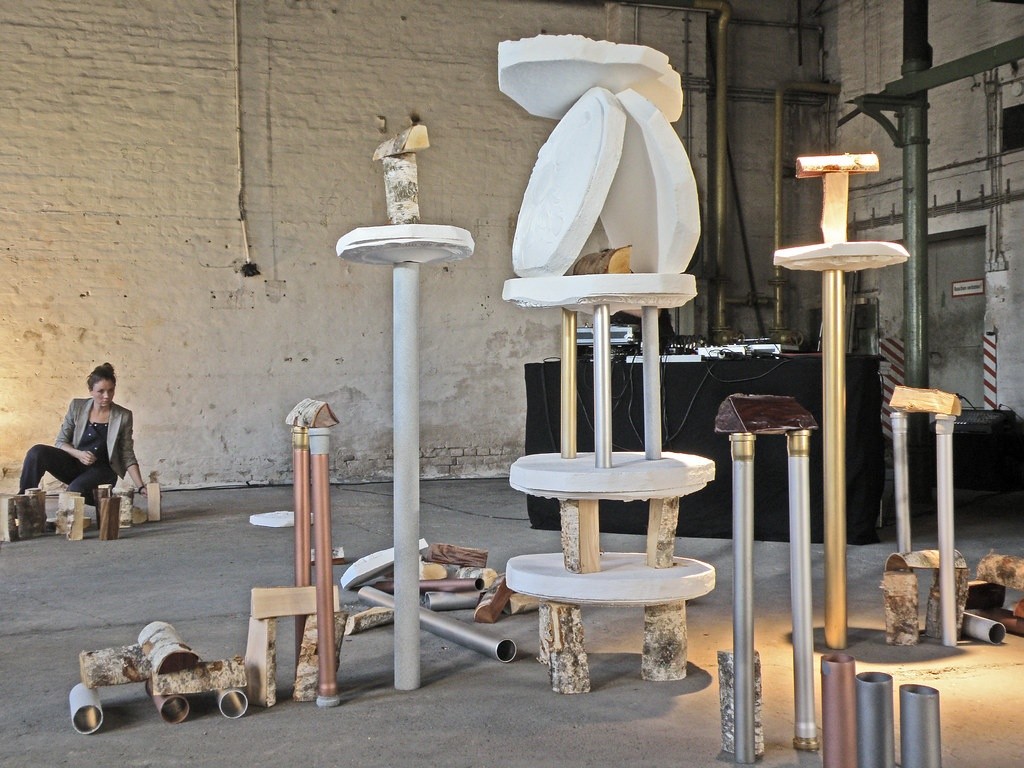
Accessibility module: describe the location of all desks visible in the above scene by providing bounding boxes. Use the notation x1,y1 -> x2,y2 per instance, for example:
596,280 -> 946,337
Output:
524,356 -> 885,545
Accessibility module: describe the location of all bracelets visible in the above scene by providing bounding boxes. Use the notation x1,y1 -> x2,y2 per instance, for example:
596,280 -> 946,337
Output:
138,485 -> 145,493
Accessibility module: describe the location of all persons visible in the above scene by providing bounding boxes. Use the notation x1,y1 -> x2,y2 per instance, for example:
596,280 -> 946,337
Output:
17,362 -> 163,506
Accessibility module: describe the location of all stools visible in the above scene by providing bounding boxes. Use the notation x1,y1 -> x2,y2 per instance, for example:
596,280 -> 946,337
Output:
505,551 -> 715,695
510,452 -> 719,576
502,272 -> 698,470
